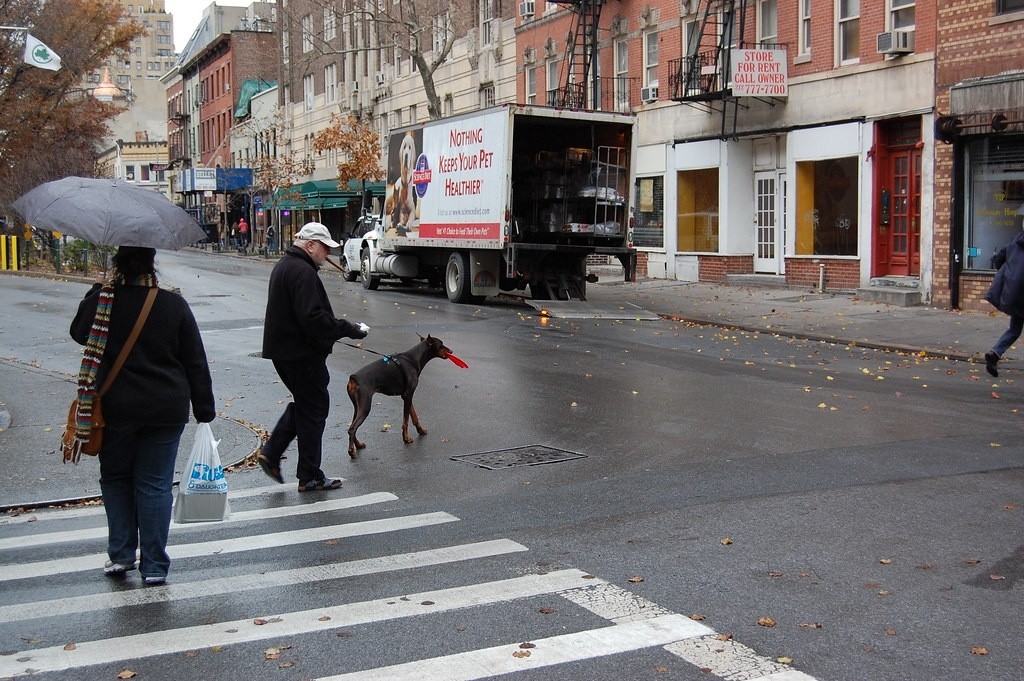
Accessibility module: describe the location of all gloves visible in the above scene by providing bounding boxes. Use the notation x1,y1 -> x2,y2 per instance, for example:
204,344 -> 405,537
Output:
349,322 -> 370,340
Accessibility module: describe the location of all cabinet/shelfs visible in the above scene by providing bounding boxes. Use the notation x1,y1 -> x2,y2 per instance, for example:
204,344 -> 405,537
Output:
530,145 -> 627,245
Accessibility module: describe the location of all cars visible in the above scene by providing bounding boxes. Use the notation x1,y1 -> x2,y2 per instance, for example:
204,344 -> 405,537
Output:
340,215 -> 383,281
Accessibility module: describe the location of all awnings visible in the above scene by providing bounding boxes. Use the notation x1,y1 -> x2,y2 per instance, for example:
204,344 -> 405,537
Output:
262,178 -> 386,210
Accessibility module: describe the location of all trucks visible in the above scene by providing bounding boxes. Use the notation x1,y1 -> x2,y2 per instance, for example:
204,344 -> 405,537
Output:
362,103 -> 639,303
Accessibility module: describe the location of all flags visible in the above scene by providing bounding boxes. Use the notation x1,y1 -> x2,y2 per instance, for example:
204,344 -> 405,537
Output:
24,33 -> 62,71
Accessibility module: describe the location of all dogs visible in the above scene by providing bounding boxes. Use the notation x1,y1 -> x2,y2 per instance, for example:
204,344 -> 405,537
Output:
386,133 -> 418,231
345,330 -> 453,460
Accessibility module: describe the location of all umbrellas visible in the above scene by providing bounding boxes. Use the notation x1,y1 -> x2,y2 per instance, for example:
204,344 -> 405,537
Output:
9,176 -> 209,279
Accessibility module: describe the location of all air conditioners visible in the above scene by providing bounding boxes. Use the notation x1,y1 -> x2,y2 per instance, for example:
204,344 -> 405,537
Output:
519,1 -> 535,17
876,30 -> 913,55
641,86 -> 658,101
226,84 -> 231,90
350,81 -> 358,92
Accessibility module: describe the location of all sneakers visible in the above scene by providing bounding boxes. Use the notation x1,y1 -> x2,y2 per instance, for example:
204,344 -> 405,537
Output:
141,572 -> 166,584
984,351 -> 998,378
104,559 -> 136,572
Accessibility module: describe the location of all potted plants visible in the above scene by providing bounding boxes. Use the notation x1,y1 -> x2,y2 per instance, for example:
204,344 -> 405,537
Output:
376,74 -> 384,84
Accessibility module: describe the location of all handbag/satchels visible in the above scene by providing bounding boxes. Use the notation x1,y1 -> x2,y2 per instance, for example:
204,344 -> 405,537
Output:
172,422 -> 231,524
63,391 -> 105,457
231,229 -> 235,235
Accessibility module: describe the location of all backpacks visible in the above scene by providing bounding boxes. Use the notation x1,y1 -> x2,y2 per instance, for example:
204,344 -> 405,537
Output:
267,227 -> 274,237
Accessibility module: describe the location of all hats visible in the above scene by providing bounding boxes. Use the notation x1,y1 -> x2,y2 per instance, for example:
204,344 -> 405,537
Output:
295,222 -> 340,248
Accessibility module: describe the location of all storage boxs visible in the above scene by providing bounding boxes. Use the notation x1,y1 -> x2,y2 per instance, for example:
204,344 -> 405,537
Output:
567,146 -> 592,161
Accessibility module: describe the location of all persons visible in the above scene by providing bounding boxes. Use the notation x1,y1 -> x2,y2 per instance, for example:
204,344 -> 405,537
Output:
69,245 -> 216,584
259,222 -> 367,492
266,223 -> 276,253
984,219 -> 1024,378
217,219 -> 249,250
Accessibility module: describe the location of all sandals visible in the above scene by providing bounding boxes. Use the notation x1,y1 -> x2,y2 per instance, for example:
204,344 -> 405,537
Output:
298,478 -> 342,491
258,453 -> 285,484
